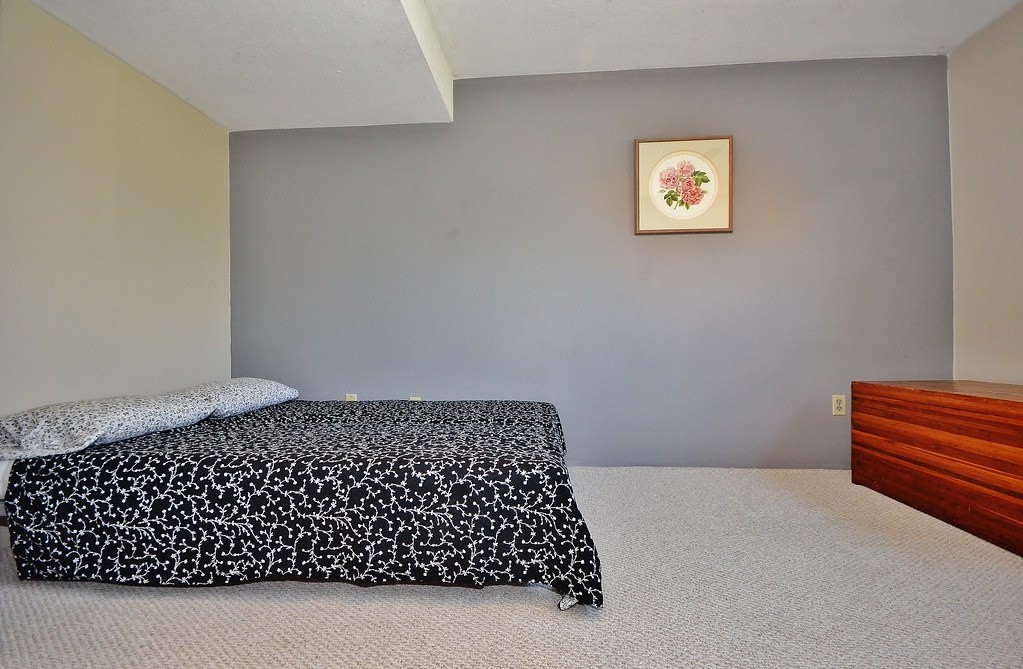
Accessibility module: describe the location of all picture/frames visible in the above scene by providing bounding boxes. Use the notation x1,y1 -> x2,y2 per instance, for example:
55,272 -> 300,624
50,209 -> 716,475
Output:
634,134 -> 734,234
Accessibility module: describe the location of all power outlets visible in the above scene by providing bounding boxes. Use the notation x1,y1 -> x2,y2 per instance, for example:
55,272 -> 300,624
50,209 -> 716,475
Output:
832,395 -> 845,415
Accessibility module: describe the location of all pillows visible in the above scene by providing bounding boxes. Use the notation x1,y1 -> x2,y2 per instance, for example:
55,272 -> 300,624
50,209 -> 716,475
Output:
0,398 -> 218,462
164,377 -> 300,420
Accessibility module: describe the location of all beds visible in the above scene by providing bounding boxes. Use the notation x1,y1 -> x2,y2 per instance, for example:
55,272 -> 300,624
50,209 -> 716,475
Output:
0,399 -> 605,609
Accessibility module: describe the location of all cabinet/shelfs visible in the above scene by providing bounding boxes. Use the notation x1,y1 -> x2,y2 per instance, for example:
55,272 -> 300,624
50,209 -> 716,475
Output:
851,380 -> 1023,559
